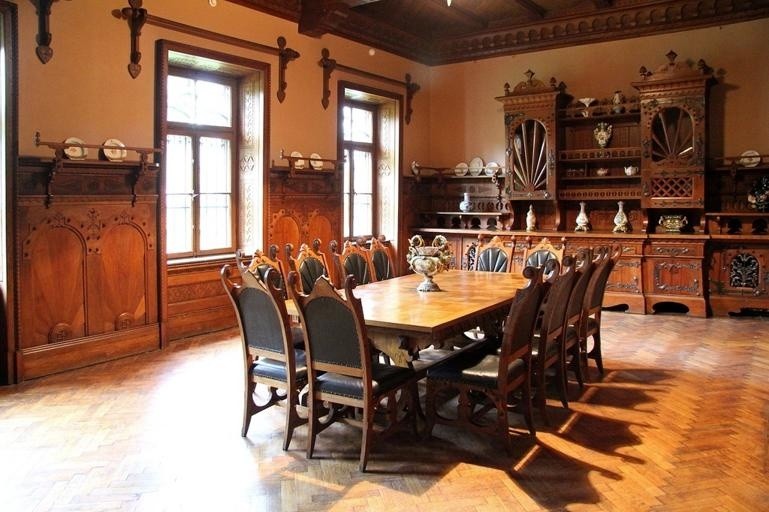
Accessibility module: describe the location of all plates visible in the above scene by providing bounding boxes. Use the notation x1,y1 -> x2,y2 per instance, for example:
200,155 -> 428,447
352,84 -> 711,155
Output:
740,151 -> 760,168
103,139 -> 128,163
454,157 -> 499,176
290,151 -> 305,170
64,138 -> 88,161
310,154 -> 323,170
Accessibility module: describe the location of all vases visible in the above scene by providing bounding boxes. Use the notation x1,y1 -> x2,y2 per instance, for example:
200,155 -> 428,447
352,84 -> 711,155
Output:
459,191 -> 475,212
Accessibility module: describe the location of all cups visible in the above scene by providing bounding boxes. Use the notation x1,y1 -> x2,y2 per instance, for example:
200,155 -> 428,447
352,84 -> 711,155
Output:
693,226 -> 703,232
596,168 -> 609,177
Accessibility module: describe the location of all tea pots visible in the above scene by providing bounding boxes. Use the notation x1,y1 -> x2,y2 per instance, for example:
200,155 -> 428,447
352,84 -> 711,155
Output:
624,165 -> 638,176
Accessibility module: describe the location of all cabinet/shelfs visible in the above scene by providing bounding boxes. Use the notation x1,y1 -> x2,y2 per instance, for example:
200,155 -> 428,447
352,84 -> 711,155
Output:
493,48 -> 718,210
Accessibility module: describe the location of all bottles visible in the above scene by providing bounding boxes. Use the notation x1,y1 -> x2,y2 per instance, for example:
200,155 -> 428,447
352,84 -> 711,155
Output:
459,192 -> 474,213
614,92 -> 623,113
526,205 -> 537,227
576,201 -> 589,225
614,202 -> 628,225
